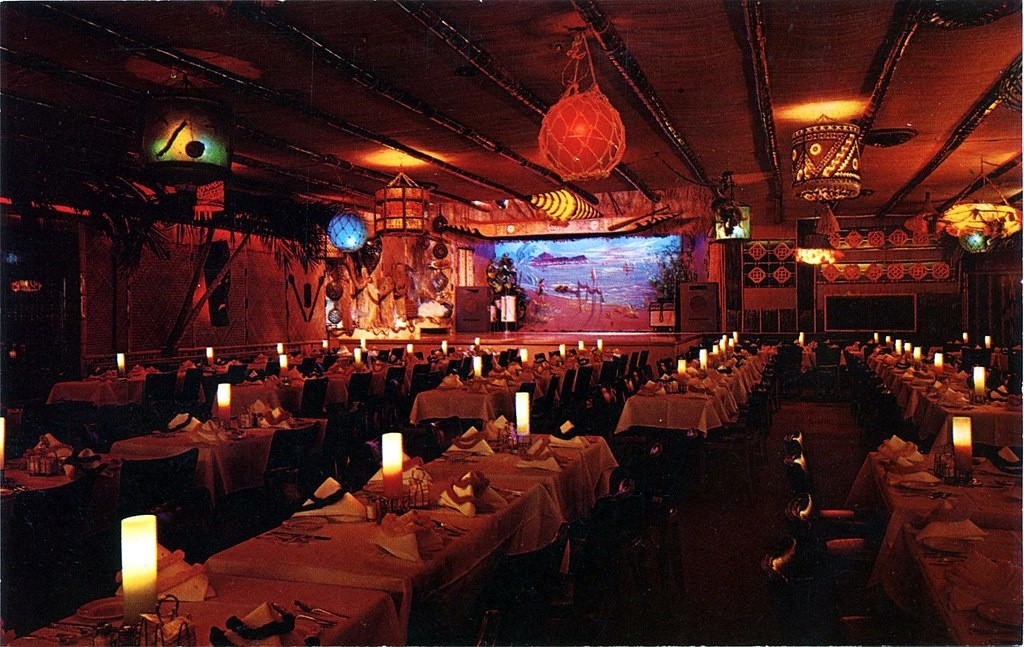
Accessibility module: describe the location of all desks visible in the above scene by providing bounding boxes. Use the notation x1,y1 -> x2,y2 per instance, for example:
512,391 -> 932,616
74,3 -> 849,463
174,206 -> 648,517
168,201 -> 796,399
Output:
421,434 -> 619,514
410,348 -> 624,439
11,570 -> 399,645
0,448 -> 174,494
113,407 -> 329,520
176,359 -> 266,386
325,349 -> 501,394
867,348 -> 1023,451
614,344 -> 776,448
210,374 -> 347,419
763,343 -> 865,371
881,528 -> 1022,647
251,347 -> 389,369
46,373 -> 212,407
203,481 -> 564,646
844,450 -> 1022,593
946,349 -> 1010,375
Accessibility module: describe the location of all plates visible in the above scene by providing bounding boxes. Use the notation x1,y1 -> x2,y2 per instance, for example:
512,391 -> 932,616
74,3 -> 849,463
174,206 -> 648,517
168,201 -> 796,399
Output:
900,480 -> 933,489
77,596 -> 124,618
282,517 -> 327,529
442,451 -> 471,457
363,485 -> 384,492
923,537 -> 976,553
976,603 -> 1023,626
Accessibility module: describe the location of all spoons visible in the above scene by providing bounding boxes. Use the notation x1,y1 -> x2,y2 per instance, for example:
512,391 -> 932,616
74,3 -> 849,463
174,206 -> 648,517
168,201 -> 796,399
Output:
902,492 -> 943,499
270,602 -> 336,624
51,621 -> 111,629
293,600 -> 349,619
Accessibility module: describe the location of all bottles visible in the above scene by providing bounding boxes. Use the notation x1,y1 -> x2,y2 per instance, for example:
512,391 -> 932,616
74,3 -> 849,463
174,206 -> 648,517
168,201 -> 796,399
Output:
231,415 -> 238,431
93,623 -> 112,647
366,496 -> 376,521
377,497 -> 388,523
117,625 -> 137,647
257,413 -> 263,426
251,413 -> 257,426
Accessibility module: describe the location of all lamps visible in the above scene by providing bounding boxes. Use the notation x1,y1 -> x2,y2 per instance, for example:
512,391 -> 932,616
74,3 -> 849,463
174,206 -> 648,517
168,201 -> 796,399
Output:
940,173 -> 1023,238
323,339 -> 328,350
973,368 -> 985,398
475,337 -> 480,348
120,515 -> 159,623
139,81 -> 234,186
792,118 -> 860,236
720,339 -> 725,357
217,383 -> 231,423
934,352 -> 942,374
677,359 -> 686,385
442,340 -> 447,356
874,333 -> 878,344
914,347 -> 921,366
722,335 -> 727,345
382,433 -> 403,499
699,349 -> 707,372
407,344 -> 413,356
597,339 -> 603,354
905,343 -> 911,352
896,340 -> 902,356
560,344 -> 566,360
354,348 -> 361,365
985,335 -> 991,347
885,336 -> 891,347
277,343 -> 283,355
515,392 -> 531,437
360,338 -> 366,348
729,338 -> 734,352
715,203 -> 751,242
473,356 -> 481,381
799,331 -> 804,347
963,332 -> 968,343
280,355 -> 287,377
713,345 -> 719,366
579,340 -> 584,353
207,348 -> 213,367
0,417 -> 5,471
521,349 -> 527,368
733,331 -> 738,344
952,416 -> 972,471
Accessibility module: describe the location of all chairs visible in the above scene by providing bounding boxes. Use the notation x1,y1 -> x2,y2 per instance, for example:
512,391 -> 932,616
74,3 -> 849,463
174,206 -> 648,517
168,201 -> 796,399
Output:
0,464 -> 108,638
762,536 -> 893,647
475,520 -> 621,647
564,488 -> 686,608
242,421 -> 321,532
618,444 -> 691,589
786,495 -> 904,572
322,403 -> 365,471
116,448 -> 213,548
426,415 -> 462,459
783,431 -> 886,529
38,330 -> 1021,442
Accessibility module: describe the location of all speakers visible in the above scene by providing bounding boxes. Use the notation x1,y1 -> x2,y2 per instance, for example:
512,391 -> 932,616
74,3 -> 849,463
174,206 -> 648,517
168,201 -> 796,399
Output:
455,285 -> 491,333
650,302 -> 677,327
679,282 -> 720,332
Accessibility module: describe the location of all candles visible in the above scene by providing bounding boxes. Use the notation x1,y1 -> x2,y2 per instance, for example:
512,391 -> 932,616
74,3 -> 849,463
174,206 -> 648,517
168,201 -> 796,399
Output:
117,353 -> 125,377
